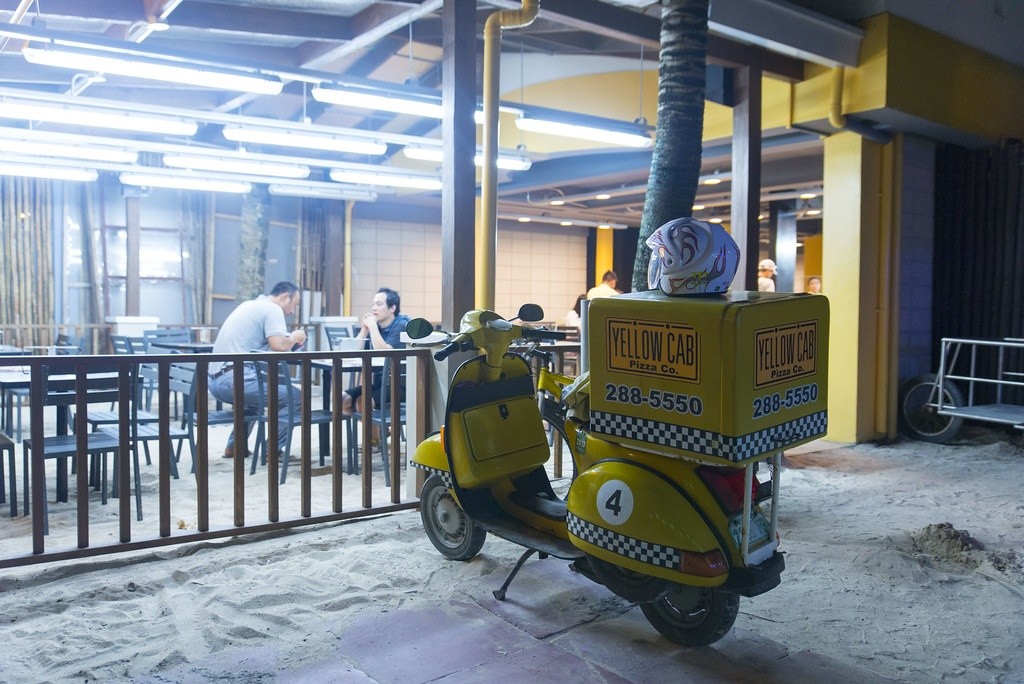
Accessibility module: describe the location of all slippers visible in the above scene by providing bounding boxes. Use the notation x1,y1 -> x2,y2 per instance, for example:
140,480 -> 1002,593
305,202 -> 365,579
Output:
265,451 -> 301,462
222,451 -> 252,458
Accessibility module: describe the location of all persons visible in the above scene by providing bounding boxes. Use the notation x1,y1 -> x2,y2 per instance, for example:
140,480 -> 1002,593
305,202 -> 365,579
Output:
207,281 -> 306,461
807,276 -> 822,295
565,294 -> 587,356
586,272 -> 621,300
342,286 -> 413,452
758,258 -> 779,292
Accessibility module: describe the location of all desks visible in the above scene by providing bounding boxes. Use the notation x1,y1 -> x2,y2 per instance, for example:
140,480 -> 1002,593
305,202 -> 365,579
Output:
149,341 -> 223,415
23,345 -> 80,356
1,365 -> 144,442
508,326 -> 580,479
298,352 -> 406,465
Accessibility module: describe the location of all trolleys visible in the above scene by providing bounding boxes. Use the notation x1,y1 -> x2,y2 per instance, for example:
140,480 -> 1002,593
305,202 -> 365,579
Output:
898,334 -> 1024,448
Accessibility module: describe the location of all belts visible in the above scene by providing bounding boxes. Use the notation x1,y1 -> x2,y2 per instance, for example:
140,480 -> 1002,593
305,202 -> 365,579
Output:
208,366 -> 234,379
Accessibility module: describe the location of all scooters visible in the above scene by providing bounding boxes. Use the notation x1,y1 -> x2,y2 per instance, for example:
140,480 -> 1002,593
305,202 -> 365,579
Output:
403,286 -> 834,649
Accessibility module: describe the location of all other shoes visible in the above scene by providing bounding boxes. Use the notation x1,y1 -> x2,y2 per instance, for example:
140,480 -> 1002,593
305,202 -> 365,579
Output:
358,440 -> 382,453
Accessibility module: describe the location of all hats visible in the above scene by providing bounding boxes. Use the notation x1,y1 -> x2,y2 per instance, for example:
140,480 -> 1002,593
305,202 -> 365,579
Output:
759,259 -> 778,275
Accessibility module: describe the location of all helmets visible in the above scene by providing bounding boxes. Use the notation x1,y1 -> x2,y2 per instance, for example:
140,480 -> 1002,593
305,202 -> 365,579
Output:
645,217 -> 740,295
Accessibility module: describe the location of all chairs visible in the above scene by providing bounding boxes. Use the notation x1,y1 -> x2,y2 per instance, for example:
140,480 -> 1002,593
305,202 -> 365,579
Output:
0,311 -> 576,541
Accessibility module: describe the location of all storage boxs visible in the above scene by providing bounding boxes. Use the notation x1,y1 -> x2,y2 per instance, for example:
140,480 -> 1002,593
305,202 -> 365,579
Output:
579,287 -> 830,467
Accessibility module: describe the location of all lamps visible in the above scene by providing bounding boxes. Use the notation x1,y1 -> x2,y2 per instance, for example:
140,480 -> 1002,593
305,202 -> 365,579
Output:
307,14 -> 501,119
0,136 -> 141,163
20,41 -> 284,95
164,154 -> 312,176
514,41 -> 659,148
0,96 -> 198,137
329,168 -> 443,189
0,160 -> 107,188
218,96 -> 388,154
116,167 -> 249,193
267,181 -> 381,201
402,146 -> 533,170
